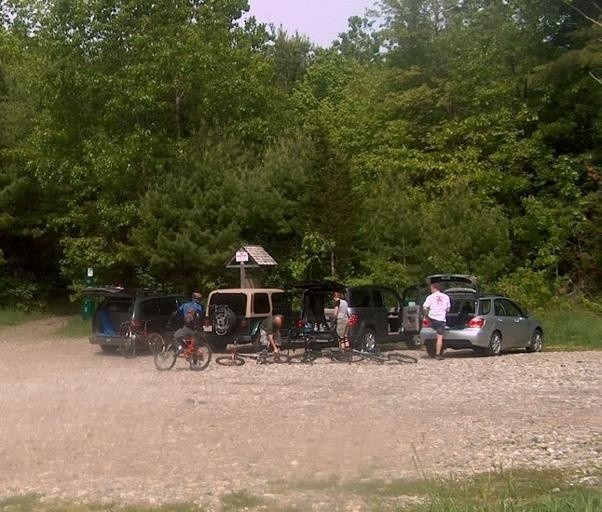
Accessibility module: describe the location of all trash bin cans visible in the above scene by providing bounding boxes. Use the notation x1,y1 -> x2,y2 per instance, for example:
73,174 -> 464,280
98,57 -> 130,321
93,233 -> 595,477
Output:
81,300 -> 94,321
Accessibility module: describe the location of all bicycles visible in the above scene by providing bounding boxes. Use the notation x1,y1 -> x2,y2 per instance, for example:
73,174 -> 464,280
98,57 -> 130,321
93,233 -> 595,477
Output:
119,319 -> 163,358
155,323 -> 211,370
216,334 -> 417,367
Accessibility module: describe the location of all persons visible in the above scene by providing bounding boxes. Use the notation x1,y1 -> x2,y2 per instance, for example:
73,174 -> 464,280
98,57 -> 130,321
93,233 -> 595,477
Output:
171,292 -> 204,356
331,290 -> 351,351
421,282 -> 452,362
258,313 -> 286,353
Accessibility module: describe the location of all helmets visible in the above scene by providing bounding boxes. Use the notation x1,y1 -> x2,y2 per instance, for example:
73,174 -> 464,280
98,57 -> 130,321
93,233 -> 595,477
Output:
192,293 -> 202,299
